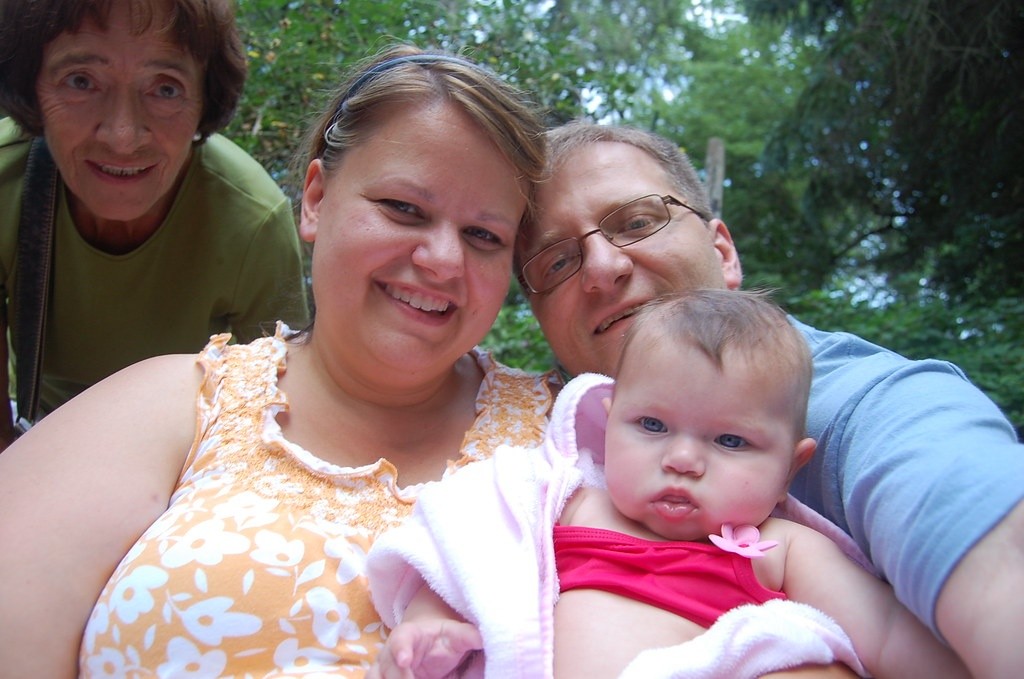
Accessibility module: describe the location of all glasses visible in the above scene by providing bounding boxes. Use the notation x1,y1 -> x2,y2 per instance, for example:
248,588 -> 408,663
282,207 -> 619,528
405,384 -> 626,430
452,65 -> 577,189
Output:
523,194 -> 711,295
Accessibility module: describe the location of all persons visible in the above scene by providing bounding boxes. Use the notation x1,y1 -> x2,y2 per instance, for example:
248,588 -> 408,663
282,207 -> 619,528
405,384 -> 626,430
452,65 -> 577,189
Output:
365,290 -> 968,679
0,47 -> 563,678
0,0 -> 311,450
513,126 -> 1024,679
